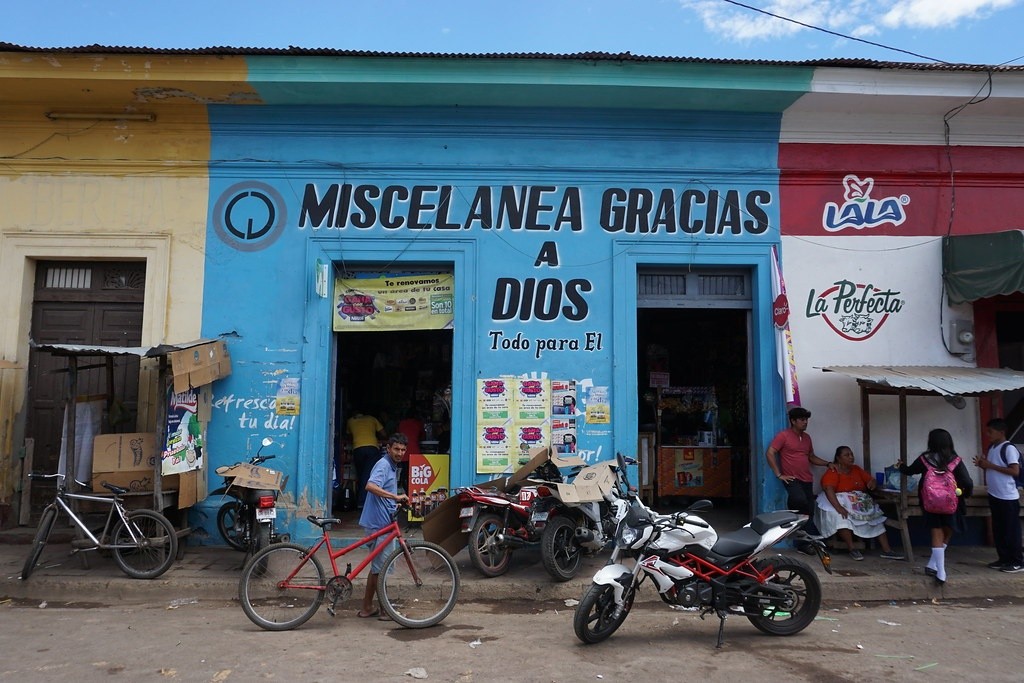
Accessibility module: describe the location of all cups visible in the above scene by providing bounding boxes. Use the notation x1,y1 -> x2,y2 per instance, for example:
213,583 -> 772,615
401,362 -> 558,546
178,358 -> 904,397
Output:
876,473 -> 884,486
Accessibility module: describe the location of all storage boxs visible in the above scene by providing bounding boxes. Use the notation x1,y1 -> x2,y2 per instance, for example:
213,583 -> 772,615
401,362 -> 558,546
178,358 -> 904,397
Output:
93,431 -> 173,493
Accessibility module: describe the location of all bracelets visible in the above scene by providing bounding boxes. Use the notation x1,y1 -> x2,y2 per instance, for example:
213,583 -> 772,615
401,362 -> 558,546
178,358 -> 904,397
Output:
827,462 -> 832,467
776,473 -> 782,478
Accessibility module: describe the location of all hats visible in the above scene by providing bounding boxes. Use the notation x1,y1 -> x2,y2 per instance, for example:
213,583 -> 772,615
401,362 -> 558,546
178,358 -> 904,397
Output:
786,408 -> 811,418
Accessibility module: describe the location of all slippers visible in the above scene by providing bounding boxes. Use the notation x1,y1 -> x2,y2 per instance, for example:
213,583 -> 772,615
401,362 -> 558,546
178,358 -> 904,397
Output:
357,607 -> 385,617
378,611 -> 407,621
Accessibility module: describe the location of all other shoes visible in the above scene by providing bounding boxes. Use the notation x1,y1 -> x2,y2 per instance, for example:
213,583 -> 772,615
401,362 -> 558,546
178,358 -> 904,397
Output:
925,567 -> 937,576
880,550 -> 904,559
935,576 -> 945,586
797,544 -> 817,557
849,549 -> 864,561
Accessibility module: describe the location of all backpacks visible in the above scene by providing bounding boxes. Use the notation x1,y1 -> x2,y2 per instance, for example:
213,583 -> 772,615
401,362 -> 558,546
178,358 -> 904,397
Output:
988,441 -> 1024,490
920,454 -> 961,514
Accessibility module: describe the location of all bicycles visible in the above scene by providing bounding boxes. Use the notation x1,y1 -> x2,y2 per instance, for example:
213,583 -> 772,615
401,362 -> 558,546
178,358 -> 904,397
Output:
21,474 -> 179,580
237,500 -> 461,632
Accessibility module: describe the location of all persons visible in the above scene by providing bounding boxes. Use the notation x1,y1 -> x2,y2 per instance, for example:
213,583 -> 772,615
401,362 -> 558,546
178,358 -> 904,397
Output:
897,428 -> 974,582
399,408 -> 426,511
346,412 -> 388,507
972,417 -> 1024,573
357,433 -> 409,621
377,411 -> 395,440
432,423 -> 446,452
766,407 -> 839,556
820,445 -> 904,560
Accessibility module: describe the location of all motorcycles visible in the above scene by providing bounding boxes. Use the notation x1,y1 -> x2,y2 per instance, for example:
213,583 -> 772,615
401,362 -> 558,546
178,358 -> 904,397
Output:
572,450 -> 834,649
448,445 -> 641,584
215,435 -> 291,575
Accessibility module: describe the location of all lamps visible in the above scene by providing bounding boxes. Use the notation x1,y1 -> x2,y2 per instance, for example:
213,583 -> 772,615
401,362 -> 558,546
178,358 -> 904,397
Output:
43,112 -> 156,122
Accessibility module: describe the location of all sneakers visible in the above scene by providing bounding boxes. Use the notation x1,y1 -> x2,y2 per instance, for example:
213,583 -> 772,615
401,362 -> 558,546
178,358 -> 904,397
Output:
1000,560 -> 1024,573
988,559 -> 1012,569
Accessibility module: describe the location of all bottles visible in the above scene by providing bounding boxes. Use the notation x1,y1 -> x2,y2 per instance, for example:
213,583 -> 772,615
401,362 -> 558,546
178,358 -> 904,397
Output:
674,464 -> 704,488
425,416 -> 433,441
411,487 -> 448,518
343,447 -> 358,507
710,448 -> 720,467
